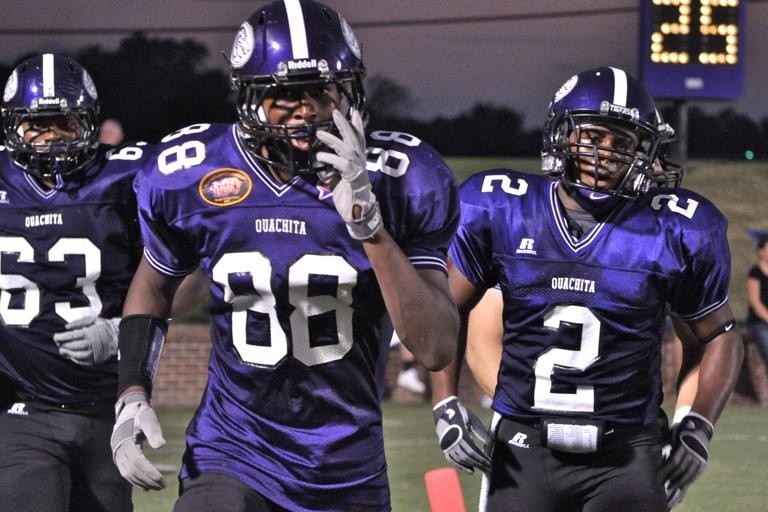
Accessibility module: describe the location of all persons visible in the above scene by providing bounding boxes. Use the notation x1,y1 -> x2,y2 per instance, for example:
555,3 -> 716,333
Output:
745,236 -> 768,373
109,0 -> 462,512
428,66 -> 745,512
462,101 -> 704,512
0,53 -> 210,512
389,330 -> 427,395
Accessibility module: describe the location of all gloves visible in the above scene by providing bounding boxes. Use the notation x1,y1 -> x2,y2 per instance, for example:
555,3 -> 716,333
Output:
54,316 -> 122,365
656,414 -> 712,508
315,108 -> 385,240
110,392 -> 168,492
432,395 -> 491,473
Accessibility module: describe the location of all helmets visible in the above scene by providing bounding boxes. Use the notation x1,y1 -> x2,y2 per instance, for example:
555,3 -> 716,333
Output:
541,69 -> 684,201
1,53 -> 102,191
230,0 -> 367,176
